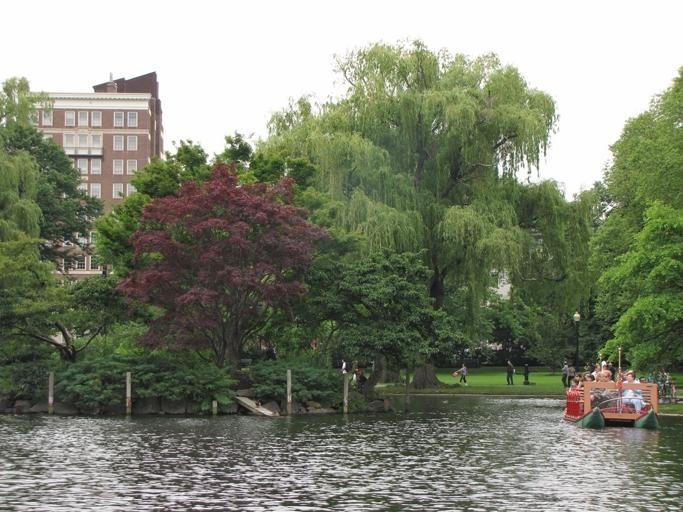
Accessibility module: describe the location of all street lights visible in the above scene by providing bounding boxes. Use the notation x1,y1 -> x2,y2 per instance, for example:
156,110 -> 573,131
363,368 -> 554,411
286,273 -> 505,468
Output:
573,308 -> 581,372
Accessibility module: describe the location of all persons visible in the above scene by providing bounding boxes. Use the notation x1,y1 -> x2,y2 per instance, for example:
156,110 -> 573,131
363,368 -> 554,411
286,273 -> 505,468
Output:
567,359 -> 675,414
504,359 -> 514,386
560,360 -> 568,388
341,358 -> 368,391
456,362 -> 469,385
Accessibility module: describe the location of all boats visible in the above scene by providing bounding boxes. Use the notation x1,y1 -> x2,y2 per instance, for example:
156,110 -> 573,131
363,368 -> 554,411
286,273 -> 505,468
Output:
562,346 -> 659,428
